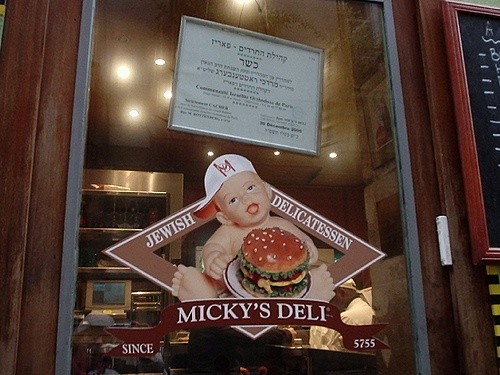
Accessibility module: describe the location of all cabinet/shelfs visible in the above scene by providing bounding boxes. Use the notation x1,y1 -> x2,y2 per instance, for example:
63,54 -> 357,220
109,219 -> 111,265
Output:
74,168 -> 183,326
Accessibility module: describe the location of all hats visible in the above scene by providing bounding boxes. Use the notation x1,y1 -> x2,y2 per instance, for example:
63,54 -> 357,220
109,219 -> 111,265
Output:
193,154 -> 257,219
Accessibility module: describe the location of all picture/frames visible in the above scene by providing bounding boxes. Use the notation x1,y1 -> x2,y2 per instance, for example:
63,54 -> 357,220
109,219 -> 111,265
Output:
441,0 -> 500,267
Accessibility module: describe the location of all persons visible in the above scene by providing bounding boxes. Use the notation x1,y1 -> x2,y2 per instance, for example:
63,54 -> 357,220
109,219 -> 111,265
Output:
309,279 -> 374,348
172,154 -> 335,302
170,277 -> 298,375
71,313 -> 118,375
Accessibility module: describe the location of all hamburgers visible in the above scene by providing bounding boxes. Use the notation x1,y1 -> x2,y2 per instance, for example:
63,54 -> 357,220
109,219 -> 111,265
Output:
237,226 -> 311,299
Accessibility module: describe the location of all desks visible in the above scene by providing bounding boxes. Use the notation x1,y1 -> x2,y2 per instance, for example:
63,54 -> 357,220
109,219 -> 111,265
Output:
264,343 -> 377,375
